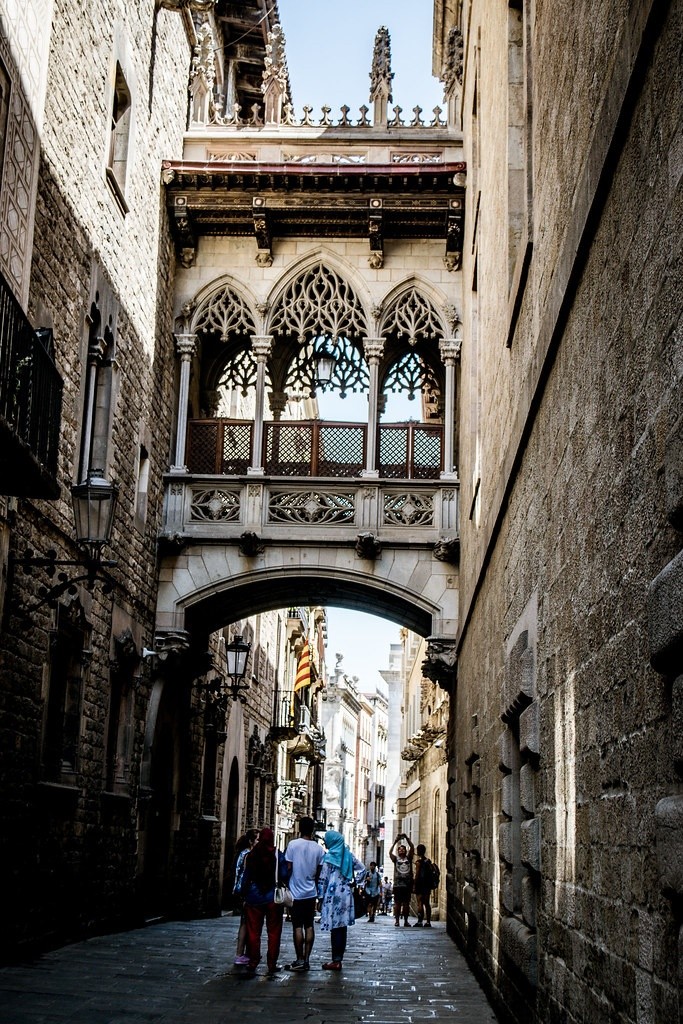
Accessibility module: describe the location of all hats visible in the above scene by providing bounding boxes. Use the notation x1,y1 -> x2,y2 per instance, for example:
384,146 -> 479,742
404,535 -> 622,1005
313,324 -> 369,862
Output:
257,828 -> 273,841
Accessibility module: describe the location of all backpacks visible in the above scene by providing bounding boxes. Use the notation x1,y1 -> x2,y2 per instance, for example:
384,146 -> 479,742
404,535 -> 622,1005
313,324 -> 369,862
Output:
420,861 -> 440,890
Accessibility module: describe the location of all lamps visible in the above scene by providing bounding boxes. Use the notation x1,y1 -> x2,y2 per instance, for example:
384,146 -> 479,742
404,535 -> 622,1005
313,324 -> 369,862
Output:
313,802 -> 326,830
273,756 -> 311,790
12,468 -> 120,590
190,635 -> 250,702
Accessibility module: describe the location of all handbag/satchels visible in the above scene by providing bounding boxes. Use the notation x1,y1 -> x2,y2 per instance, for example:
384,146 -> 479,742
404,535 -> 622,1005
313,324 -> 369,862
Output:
352,888 -> 368,919
273,884 -> 294,908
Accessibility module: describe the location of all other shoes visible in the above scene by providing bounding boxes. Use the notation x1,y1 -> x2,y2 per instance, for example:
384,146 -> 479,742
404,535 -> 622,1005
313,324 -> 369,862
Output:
423,923 -> 431,927
367,919 -> 374,923
269,966 -> 281,973
395,922 -> 399,926
413,923 -> 423,927
322,961 -> 342,971
285,960 -> 309,972
234,954 -> 250,964
404,923 -> 411,927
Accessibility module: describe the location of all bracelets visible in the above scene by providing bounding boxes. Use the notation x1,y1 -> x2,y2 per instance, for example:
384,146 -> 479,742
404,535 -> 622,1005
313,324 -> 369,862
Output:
406,838 -> 410,840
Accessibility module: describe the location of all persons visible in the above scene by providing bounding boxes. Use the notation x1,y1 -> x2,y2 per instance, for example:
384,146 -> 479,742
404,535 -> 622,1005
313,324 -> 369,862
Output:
232,817 -> 365,970
413,844 -> 433,927
390,833 -> 414,927
354,862 -> 392,922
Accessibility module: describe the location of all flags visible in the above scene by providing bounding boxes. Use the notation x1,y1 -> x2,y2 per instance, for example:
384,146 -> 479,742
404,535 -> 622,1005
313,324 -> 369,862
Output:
290,639 -> 310,727
